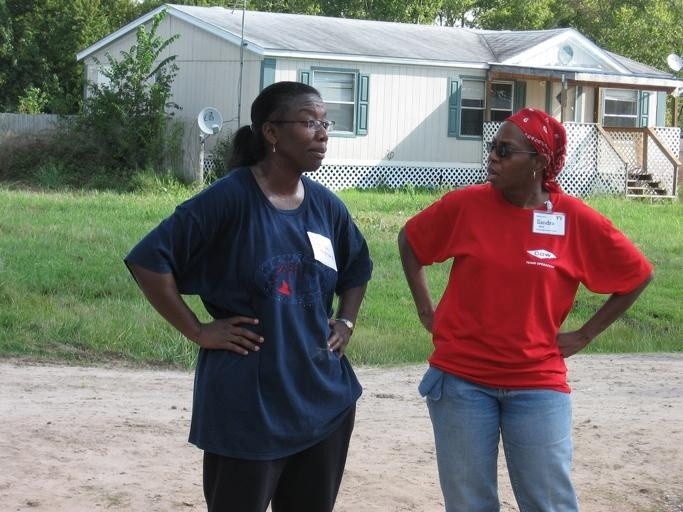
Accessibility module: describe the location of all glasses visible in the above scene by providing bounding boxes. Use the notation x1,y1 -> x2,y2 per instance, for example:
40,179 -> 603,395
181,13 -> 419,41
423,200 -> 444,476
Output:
265,117 -> 337,133
485,140 -> 537,158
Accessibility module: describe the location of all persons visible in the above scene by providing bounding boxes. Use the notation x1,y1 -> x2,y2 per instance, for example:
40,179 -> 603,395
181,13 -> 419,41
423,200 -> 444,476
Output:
396,104 -> 658,512
118,79 -> 376,512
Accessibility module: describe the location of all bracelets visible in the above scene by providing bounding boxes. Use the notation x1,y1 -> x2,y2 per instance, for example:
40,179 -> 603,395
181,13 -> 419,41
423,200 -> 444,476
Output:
335,317 -> 354,336
